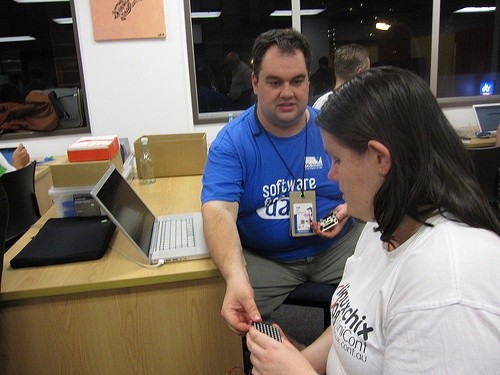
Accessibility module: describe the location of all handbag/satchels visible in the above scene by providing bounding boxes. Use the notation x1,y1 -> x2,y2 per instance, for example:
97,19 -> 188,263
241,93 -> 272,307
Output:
31,88 -> 83,130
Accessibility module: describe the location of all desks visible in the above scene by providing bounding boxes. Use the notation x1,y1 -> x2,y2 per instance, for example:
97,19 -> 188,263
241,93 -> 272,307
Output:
456,123 -> 500,172
0,176 -> 245,374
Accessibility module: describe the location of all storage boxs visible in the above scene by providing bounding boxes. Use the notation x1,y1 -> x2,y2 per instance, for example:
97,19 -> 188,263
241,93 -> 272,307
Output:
135,132 -> 208,178
47,184 -> 109,218
50,152 -> 124,184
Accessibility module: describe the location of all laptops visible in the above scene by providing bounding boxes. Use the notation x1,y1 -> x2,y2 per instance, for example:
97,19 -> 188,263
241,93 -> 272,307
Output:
473,103 -> 500,134
90,164 -> 211,265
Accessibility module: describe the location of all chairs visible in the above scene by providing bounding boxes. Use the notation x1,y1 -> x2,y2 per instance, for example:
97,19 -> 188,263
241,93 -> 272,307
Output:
0,160 -> 44,253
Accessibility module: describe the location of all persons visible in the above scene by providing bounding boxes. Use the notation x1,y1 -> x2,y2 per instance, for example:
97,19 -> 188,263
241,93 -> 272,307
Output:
202,29 -> 367,352
197,52 -> 336,114
312,43 -> 370,110
246,66 -> 500,374
0,144 -> 30,173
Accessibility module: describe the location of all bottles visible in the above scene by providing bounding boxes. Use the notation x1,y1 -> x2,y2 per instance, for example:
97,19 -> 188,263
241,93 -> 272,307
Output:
138,137 -> 156,185
